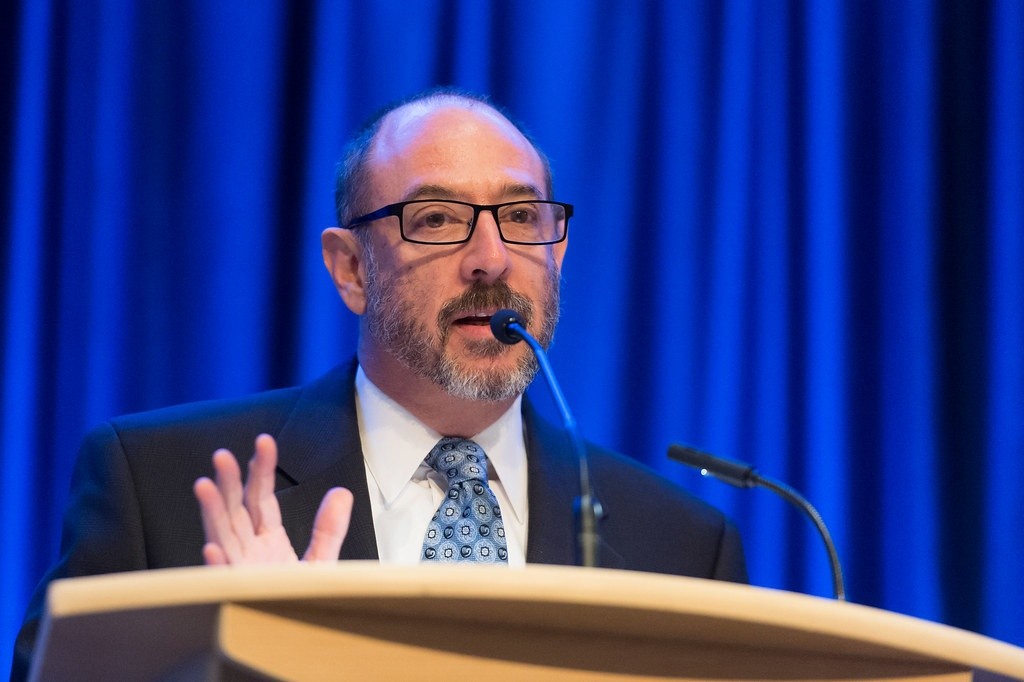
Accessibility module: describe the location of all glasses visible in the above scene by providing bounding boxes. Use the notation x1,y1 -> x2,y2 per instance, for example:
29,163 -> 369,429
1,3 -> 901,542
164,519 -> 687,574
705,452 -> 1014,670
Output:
344,201 -> 575,245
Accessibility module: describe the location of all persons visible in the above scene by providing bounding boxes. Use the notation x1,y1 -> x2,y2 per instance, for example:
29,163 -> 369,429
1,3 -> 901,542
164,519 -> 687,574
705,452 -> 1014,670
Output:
7,94 -> 751,682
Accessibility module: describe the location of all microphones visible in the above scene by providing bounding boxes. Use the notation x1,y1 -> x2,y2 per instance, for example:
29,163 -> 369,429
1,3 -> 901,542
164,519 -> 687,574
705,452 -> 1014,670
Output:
666,441 -> 846,601
491,308 -> 595,569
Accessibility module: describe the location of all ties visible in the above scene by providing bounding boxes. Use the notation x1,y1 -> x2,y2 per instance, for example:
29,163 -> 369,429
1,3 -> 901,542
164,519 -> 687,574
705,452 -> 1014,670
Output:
424,435 -> 507,564
